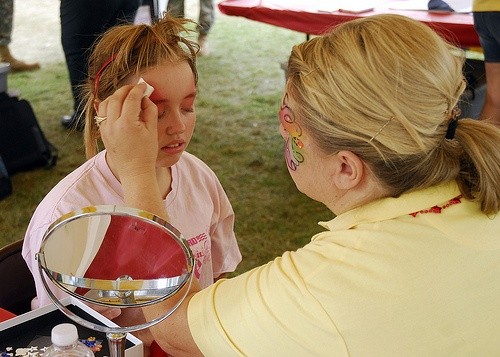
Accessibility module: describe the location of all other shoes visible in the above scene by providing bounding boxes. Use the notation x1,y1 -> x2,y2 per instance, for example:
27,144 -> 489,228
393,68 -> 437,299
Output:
198,40 -> 208,56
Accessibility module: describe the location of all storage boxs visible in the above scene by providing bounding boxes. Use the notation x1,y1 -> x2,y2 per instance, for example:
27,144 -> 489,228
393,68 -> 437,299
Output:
0,296 -> 144,357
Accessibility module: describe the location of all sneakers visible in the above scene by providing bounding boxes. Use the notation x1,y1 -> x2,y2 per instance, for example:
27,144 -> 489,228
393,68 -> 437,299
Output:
0,46 -> 40,71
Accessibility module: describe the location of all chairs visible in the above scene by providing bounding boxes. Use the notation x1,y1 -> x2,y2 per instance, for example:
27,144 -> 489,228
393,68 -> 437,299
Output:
0,239 -> 37,319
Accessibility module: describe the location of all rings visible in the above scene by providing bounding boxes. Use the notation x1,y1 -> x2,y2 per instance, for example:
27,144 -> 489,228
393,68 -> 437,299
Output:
94,117 -> 107,125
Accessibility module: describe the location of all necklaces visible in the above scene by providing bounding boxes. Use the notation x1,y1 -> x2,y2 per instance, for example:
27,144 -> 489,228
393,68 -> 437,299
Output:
408,194 -> 465,218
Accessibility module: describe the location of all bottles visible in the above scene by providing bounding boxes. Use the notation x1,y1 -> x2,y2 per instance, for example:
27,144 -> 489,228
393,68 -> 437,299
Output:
43,323 -> 96,357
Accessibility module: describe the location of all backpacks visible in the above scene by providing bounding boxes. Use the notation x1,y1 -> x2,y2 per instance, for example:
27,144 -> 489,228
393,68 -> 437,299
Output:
0,91 -> 59,175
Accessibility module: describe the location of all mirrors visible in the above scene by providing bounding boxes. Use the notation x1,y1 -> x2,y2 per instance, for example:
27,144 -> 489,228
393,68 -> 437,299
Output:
35,204 -> 199,357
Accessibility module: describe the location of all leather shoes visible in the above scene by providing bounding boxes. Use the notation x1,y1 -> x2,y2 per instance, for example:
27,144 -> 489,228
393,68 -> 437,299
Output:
62,115 -> 85,132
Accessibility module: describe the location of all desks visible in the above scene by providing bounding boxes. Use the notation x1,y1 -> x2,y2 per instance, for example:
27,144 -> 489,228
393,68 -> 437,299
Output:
218,0 -> 482,54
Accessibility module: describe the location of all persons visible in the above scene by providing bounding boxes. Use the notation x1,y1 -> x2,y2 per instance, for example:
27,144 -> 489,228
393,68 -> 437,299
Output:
0,0 -> 40,72
59,0 -> 140,131
21,11 -> 242,357
473,0 -> 500,128
166,0 -> 215,57
98,14 -> 500,357
